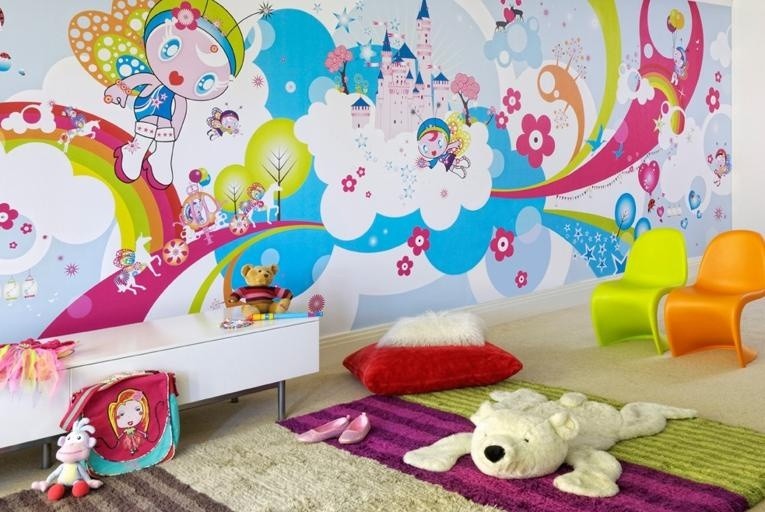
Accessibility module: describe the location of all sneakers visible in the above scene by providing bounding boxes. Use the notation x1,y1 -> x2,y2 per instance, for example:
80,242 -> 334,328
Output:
339,412 -> 371,444
299,415 -> 350,442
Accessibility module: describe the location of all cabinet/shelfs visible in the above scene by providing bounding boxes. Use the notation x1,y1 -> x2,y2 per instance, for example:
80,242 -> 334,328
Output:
0,308 -> 320,468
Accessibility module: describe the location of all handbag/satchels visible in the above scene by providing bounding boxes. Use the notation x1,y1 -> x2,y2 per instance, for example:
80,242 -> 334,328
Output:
72,371 -> 179,475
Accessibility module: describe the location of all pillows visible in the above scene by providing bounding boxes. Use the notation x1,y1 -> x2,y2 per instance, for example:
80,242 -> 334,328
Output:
342,342 -> 522,396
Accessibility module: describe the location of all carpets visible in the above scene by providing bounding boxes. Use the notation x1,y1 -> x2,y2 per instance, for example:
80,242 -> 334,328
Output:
0,380 -> 765,512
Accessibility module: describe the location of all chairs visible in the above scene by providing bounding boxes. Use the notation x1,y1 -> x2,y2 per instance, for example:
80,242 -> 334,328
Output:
593,229 -> 765,365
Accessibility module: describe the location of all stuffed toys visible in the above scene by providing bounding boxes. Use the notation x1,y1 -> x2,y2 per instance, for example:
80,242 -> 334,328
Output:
229,264 -> 293,316
401,386 -> 699,499
31,417 -> 105,501
